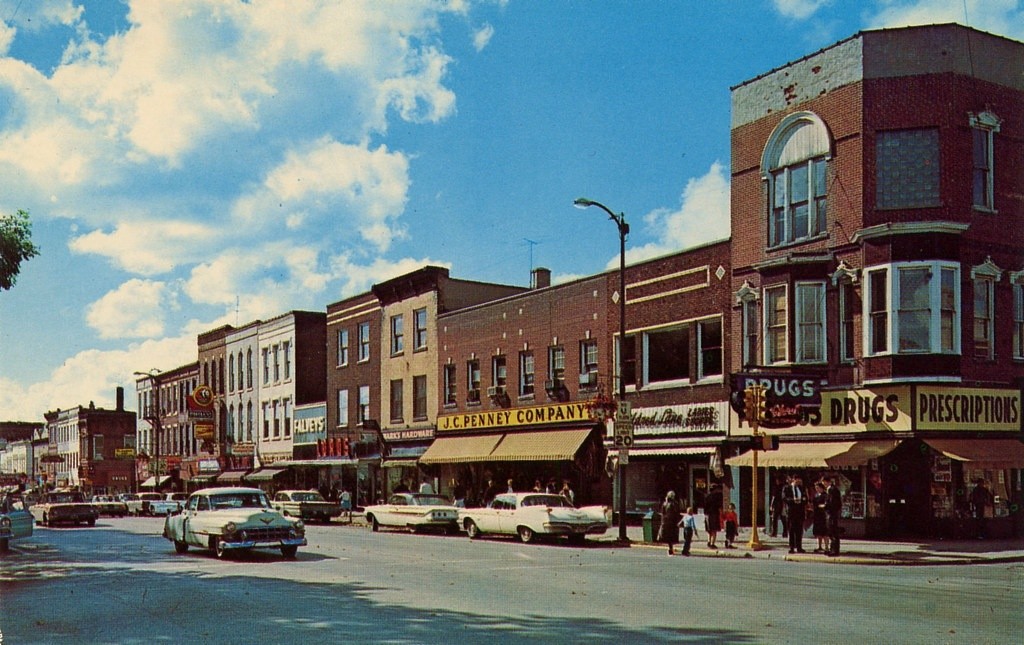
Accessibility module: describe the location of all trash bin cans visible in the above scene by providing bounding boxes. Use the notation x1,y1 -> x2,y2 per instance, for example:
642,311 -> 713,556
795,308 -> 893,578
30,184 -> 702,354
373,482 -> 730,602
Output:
642,511 -> 662,543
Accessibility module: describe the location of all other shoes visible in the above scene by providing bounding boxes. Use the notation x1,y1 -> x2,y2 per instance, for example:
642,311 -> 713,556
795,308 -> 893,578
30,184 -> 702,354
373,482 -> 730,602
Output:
788,548 -> 794,553
725,541 -> 737,549
708,542 -> 717,549
828,550 -> 838,556
818,550 -> 829,554
797,548 -> 805,553
681,549 -> 691,556
668,550 -> 673,555
814,548 -> 822,552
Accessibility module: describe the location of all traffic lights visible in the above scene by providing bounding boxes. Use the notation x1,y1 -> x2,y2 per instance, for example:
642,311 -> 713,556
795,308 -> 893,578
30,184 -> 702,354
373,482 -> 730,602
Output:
738,387 -> 754,421
757,388 -> 771,421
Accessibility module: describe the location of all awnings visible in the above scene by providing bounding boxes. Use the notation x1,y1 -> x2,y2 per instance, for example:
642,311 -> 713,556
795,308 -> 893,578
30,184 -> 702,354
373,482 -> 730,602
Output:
724,438 -> 905,468
921,438 -> 1024,470
419,428 -> 593,464
140,475 -> 172,486
381,458 -> 419,468
605,445 -> 725,478
190,469 -> 285,481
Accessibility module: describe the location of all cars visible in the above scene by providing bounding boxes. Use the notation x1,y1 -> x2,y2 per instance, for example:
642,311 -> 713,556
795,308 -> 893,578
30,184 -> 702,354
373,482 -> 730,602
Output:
162,486 -> 308,560
364,492 -> 459,535
0,496 -> 33,550
459,492 -> 611,543
269,490 -> 339,524
137,492 -> 180,515
28,493 -> 99,528
118,494 -> 142,515
87,494 -> 127,518
165,492 -> 196,509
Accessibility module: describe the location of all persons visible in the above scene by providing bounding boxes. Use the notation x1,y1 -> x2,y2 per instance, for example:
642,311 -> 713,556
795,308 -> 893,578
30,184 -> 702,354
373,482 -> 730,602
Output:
703,483 -> 738,549
656,490 -> 699,556
318,481 -> 353,517
769,472 -> 810,553
533,477 -> 575,508
418,476 -> 434,504
393,478 -> 411,505
2,492 -> 16,514
452,477 -> 514,508
970,477 -> 990,540
812,475 -> 843,557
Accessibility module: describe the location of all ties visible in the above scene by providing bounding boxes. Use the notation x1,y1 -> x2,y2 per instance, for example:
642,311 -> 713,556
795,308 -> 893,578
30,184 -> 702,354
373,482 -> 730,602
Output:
794,486 -> 798,498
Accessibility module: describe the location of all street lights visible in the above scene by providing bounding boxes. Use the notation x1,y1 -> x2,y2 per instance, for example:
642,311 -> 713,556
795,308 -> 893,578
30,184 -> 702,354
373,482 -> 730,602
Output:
134,371 -> 159,491
574,198 -> 630,543
